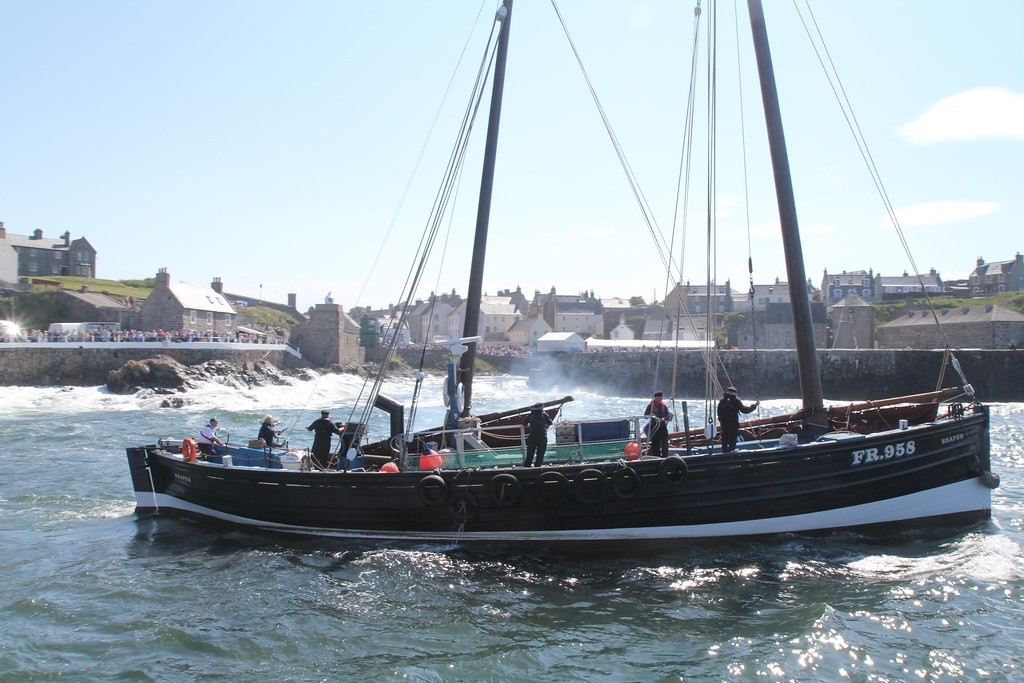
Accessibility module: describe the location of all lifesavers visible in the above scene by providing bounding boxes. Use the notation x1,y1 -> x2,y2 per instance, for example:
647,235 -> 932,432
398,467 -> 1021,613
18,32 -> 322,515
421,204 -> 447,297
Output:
443,377 -> 451,408
180,437 -> 199,463
540,473 -> 573,507
609,468 -> 645,505
456,382 -> 466,414
417,475 -> 452,509
490,475 -> 522,507
657,455 -> 690,487
575,469 -> 609,507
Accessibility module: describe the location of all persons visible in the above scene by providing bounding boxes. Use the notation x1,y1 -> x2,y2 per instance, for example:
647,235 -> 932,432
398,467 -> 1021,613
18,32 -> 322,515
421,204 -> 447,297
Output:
0,329 -> 287,344
523,402 -> 552,467
305,410 -> 344,471
198,417 -> 228,462
718,387 -> 760,452
395,343 -> 662,358
258,414 -> 284,448
644,392 -> 670,458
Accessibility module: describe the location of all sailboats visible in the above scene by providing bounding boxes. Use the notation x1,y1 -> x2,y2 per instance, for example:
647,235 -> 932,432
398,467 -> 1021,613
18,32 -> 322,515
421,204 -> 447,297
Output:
124,0 -> 995,556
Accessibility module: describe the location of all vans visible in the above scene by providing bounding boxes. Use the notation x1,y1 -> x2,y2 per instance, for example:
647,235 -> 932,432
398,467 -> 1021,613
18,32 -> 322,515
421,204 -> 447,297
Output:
49,323 -> 83,338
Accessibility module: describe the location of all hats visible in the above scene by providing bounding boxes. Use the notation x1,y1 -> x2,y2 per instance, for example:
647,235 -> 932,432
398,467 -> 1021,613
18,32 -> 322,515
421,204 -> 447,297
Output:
728,386 -> 737,394
529,403 -> 545,410
654,392 -> 663,397
321,410 -> 330,415
